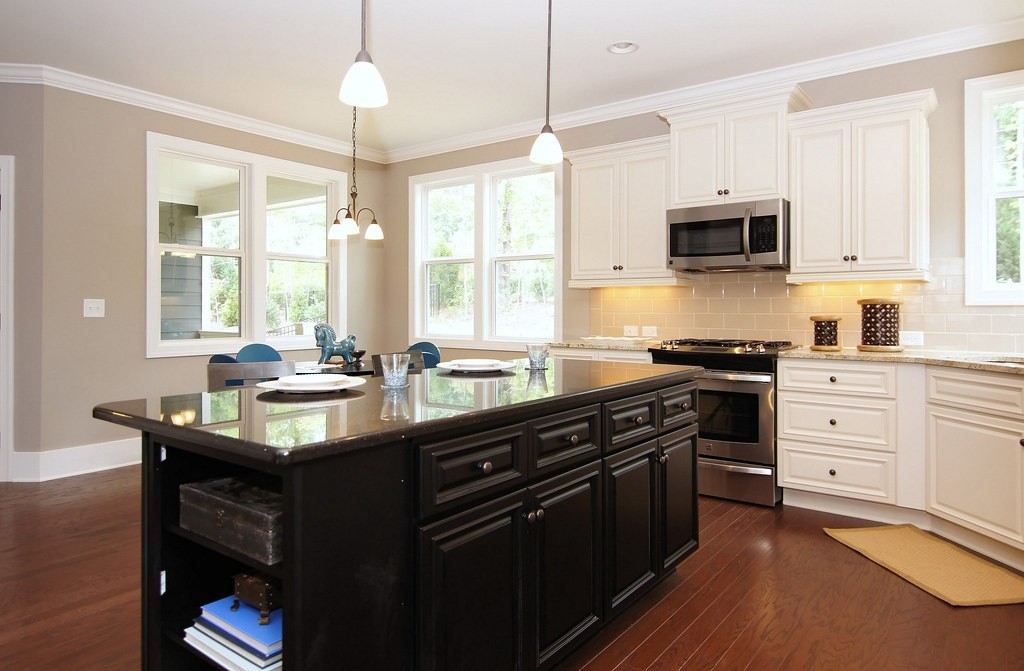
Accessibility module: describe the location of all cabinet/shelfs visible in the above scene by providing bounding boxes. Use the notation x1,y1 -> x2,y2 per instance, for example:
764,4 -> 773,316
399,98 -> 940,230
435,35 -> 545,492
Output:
772,351 -> 925,522
563,134 -> 694,288
138,379 -> 701,671
656,82 -> 814,211
784,87 -> 941,285
921,362 -> 1024,574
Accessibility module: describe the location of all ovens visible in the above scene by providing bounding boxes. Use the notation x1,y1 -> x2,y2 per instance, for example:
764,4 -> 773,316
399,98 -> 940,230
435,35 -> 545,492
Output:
695,369 -> 782,506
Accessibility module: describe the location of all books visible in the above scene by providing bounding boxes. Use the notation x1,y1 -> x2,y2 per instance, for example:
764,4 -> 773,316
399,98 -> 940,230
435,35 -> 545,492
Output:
183,595 -> 283,671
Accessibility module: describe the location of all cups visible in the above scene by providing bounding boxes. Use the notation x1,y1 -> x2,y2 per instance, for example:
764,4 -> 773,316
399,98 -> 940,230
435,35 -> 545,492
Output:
380,353 -> 410,386
526,370 -> 549,394
527,344 -> 549,368
380,390 -> 409,422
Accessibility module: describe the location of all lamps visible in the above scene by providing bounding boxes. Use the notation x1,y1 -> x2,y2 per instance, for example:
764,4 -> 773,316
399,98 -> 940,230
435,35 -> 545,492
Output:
528,0 -> 563,165
160,408 -> 197,428
159,201 -> 197,260
326,106 -> 384,241
337,0 -> 389,108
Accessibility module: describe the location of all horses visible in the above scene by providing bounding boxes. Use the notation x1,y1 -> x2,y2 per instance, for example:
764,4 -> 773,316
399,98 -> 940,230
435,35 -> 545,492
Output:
314,323 -> 356,365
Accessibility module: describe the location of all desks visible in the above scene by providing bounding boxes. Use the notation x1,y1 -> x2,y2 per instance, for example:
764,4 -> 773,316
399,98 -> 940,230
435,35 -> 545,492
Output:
296,359 -> 374,376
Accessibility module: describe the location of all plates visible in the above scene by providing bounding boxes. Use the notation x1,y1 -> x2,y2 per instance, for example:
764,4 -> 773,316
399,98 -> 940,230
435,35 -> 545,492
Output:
257,378 -> 366,393
436,362 -> 516,373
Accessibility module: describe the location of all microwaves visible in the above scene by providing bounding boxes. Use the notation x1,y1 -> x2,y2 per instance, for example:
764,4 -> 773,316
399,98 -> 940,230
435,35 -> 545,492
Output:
666,197 -> 790,273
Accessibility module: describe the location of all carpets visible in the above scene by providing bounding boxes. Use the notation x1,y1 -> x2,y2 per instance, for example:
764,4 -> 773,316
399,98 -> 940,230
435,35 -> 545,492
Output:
820,524 -> 1024,606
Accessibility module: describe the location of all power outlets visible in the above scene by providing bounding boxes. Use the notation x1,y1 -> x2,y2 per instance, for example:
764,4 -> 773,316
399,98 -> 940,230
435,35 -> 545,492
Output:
624,325 -> 639,337
901,331 -> 924,346
642,325 -> 658,336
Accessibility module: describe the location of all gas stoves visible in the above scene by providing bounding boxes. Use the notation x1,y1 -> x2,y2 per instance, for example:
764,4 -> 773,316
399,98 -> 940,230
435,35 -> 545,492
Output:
648,338 -> 793,373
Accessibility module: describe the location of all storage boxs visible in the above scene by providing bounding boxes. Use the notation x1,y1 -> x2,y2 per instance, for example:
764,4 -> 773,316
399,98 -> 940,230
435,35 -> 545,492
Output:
228,569 -> 279,617
177,477 -> 284,567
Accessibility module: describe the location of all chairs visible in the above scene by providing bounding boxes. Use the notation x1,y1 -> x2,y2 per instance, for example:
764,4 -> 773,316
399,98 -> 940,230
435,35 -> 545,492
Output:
407,341 -> 441,360
209,354 -> 244,385
207,360 -> 297,393
371,350 -> 426,376
236,343 -> 283,382
423,352 -> 440,368
